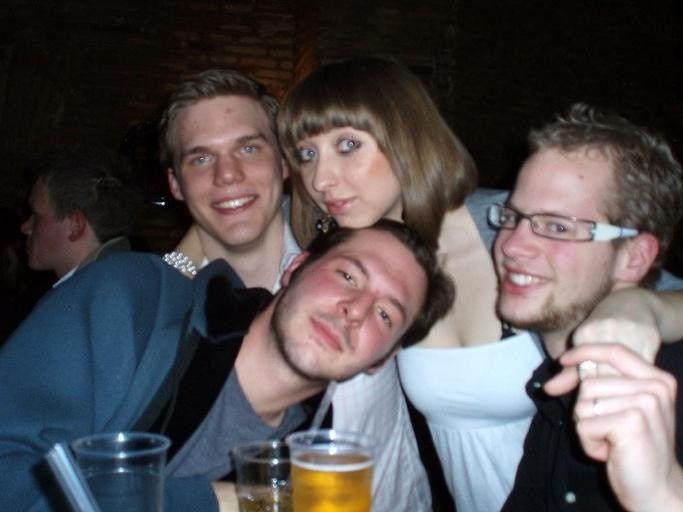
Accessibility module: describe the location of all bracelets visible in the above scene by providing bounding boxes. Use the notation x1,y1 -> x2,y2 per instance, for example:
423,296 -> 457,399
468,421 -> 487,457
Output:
161,250 -> 197,277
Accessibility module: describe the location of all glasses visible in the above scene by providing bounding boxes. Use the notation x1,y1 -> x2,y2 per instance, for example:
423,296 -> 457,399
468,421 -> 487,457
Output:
487,203 -> 639,242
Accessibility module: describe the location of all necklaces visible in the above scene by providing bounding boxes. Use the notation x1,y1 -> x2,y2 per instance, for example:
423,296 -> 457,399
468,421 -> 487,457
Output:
16,152 -> 137,288
486,99 -> 683,511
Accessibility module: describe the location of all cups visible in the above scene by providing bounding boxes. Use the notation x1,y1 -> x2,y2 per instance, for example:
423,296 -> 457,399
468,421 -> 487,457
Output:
228,440 -> 290,512
67,430 -> 172,512
282,427 -> 375,512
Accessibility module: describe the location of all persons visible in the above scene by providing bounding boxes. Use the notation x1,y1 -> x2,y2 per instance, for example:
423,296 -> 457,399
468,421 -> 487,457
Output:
163,54 -> 683,511
0,219 -> 459,510
155,67 -> 433,508
